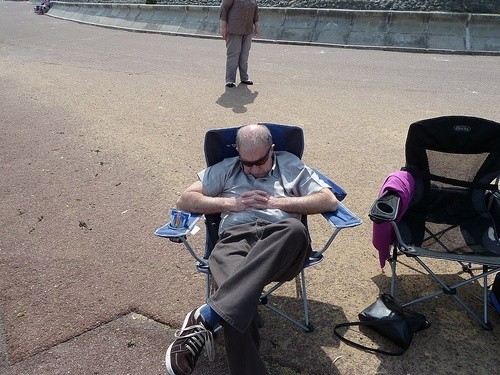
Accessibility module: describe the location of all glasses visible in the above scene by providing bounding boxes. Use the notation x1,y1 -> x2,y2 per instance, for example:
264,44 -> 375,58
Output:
238,147 -> 272,167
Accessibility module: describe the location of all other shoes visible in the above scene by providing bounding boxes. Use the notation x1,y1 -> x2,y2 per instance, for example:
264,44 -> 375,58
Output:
241,80 -> 253,85
226,82 -> 234,87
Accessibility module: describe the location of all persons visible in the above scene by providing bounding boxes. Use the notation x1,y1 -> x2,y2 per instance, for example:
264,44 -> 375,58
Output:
165,125 -> 339,375
219,0 -> 260,87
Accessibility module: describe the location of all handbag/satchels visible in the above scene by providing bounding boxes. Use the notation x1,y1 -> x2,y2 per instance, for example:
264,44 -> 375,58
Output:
334,292 -> 430,356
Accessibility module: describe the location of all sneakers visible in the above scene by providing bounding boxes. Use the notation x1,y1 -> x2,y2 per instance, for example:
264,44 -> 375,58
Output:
165,302 -> 214,375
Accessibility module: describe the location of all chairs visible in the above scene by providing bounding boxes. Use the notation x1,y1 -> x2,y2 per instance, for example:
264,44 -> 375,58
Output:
368,115 -> 500,331
156,122 -> 363,334
